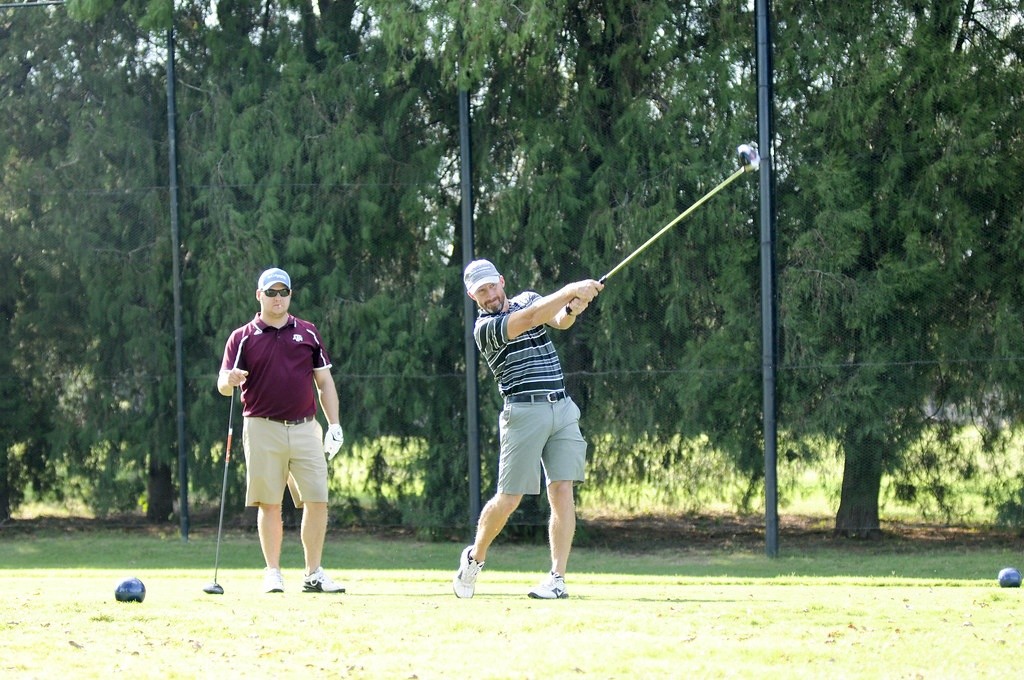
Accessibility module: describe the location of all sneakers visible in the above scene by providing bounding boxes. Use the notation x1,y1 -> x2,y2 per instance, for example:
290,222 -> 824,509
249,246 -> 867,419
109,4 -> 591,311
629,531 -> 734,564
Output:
301,567 -> 346,594
528,571 -> 570,599
263,567 -> 286,593
452,545 -> 486,599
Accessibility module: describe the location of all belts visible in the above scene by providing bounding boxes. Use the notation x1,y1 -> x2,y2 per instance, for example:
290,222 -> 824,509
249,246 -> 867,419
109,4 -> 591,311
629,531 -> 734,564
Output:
505,389 -> 569,404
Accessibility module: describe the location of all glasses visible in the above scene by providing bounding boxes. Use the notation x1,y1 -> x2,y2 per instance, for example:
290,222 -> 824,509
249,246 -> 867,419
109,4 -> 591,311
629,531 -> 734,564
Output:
264,288 -> 290,297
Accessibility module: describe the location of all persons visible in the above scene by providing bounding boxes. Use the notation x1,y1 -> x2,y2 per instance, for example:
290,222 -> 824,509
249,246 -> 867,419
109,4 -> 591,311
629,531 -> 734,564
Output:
452,259 -> 605,600
217,268 -> 348,593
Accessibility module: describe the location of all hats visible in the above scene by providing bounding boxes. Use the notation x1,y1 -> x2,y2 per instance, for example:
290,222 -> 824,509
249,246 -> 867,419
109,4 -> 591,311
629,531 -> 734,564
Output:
463,258 -> 501,295
258,267 -> 291,292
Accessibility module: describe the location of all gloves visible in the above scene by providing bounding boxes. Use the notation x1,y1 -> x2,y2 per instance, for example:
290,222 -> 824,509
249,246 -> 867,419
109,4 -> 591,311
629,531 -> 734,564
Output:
323,424 -> 344,461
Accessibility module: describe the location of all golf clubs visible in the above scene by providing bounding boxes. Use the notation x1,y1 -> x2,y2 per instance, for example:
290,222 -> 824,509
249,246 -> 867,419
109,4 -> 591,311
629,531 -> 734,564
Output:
565,144 -> 762,314
203,386 -> 240,594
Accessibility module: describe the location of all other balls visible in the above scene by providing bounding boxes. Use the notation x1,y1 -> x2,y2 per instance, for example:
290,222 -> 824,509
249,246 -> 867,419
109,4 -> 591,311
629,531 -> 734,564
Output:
115,577 -> 145,602
998,566 -> 1021,586
